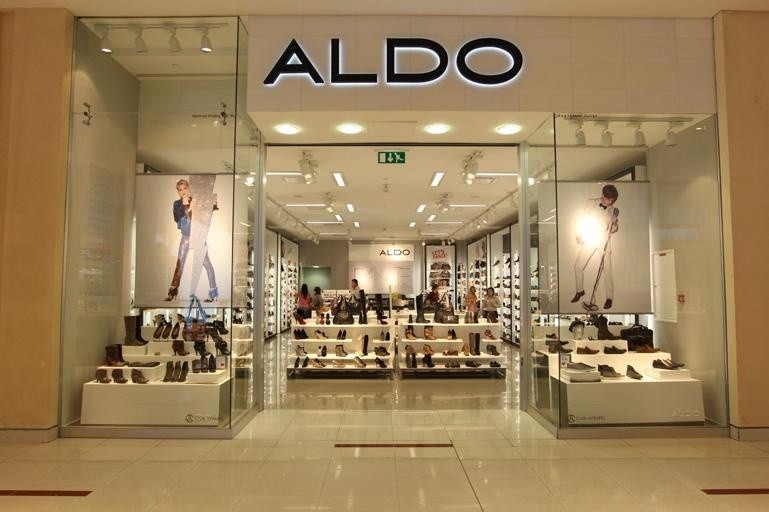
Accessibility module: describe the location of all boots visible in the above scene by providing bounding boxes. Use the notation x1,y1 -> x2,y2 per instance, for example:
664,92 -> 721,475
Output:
375,294 -> 386,319
415,294 -> 430,323
475,333 -> 480,355
358,289 -> 367,325
468,333 -> 476,355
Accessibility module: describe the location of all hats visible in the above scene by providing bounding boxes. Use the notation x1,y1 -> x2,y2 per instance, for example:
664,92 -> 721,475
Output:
597,329 -> 620,340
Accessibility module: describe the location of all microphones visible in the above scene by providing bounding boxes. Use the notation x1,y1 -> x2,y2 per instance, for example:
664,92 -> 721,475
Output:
614,208 -> 619,217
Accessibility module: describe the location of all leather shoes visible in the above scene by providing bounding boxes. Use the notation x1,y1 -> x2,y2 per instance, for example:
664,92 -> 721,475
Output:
603,345 -> 626,355
444,360 -> 501,368
668,359 -> 684,368
577,346 -> 599,355
548,344 -> 573,354
566,362 -> 596,372
625,365 -> 643,379
597,365 -> 624,380
652,359 -> 672,370
636,344 -> 660,353
570,289 -> 584,303
545,334 -> 568,345
603,299 -> 612,309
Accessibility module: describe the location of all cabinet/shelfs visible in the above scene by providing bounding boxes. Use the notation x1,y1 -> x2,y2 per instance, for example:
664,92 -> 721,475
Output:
394,322 -> 505,370
428,244 -> 456,309
284,324 -> 398,373
77,324 -> 233,429
458,215 -> 543,349
535,325 -> 709,425
290,288 -> 471,325
229,239 -> 299,374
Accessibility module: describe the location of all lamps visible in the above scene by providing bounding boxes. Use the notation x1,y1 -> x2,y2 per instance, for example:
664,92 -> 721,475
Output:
295,150 -> 336,216
86,20 -> 230,59
561,114 -> 697,150
435,150 -> 486,216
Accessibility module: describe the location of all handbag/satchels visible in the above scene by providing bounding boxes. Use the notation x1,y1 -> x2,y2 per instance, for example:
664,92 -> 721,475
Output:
346,294 -> 359,315
330,294 -> 348,316
621,323 -> 653,350
332,302 -> 353,324
180,295 -> 208,342
430,262 -> 450,288
434,292 -> 454,324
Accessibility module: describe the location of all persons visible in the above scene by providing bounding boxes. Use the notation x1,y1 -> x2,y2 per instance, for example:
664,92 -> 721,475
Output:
345,278 -> 360,309
422,284 -> 439,308
482,286 -> 500,317
158,179 -> 218,302
295,283 -> 311,319
464,286 -> 477,316
309,286 -> 323,312
569,185 -> 619,309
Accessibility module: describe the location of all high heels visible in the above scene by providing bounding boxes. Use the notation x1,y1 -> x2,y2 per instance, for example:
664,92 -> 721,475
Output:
294,314 -> 389,369
233,250 -> 299,339
162,286 -> 177,301
94,315 -> 230,384
203,287 -> 217,303
457,257 -> 540,344
213,321 -> 228,336
405,311 -> 501,369
